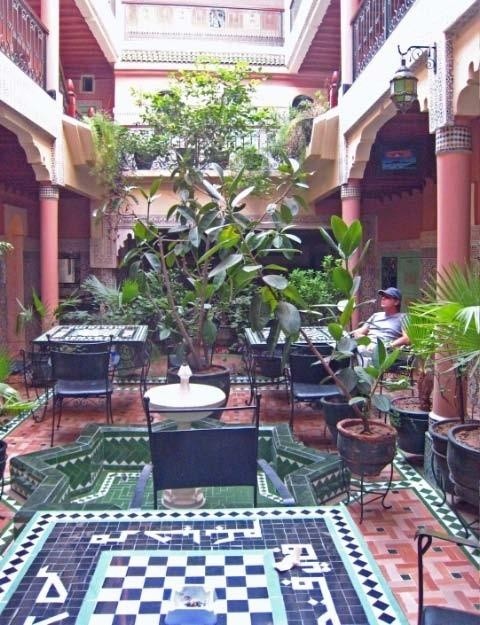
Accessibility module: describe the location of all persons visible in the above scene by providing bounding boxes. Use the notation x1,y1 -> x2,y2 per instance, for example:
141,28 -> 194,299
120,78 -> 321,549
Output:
350,287 -> 411,368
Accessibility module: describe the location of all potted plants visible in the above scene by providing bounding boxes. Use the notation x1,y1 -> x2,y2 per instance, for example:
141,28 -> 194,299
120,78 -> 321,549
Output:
386,258 -> 479,505
122,156 -> 407,479
79,53 -> 330,170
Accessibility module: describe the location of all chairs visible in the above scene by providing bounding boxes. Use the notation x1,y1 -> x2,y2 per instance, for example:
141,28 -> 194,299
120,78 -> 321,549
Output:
0,324 -> 154,445
142,392 -> 262,509
414,528 -> 480,624
240,322 -> 417,438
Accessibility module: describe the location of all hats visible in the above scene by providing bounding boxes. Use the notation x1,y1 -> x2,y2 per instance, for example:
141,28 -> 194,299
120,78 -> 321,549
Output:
378,286 -> 402,299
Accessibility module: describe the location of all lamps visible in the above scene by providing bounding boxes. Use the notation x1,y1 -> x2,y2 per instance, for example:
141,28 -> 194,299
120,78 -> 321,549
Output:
389,43 -> 437,116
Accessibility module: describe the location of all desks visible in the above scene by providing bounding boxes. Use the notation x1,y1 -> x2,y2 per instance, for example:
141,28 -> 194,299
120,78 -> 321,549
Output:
142,379 -> 225,510
30,320 -> 152,422
